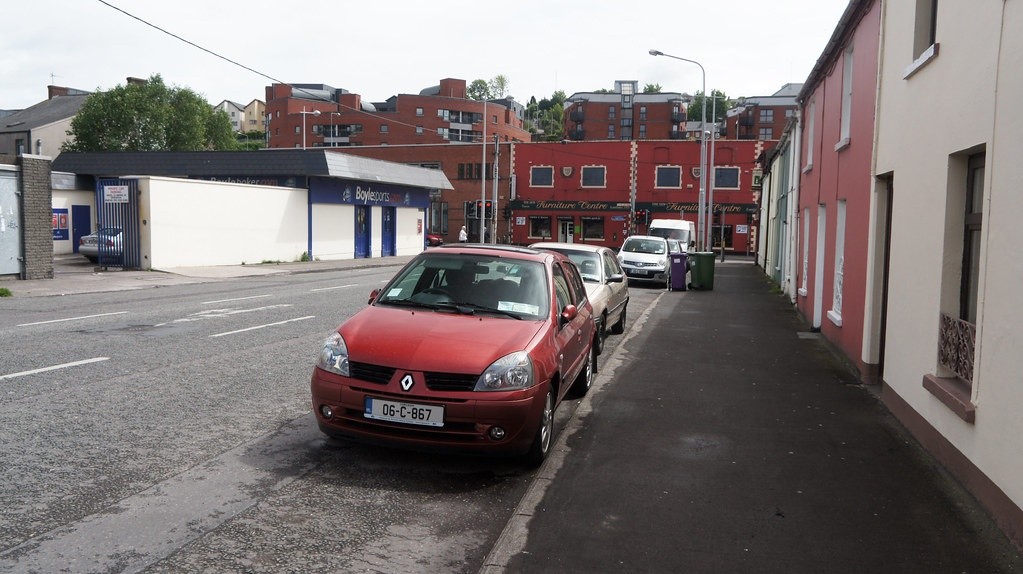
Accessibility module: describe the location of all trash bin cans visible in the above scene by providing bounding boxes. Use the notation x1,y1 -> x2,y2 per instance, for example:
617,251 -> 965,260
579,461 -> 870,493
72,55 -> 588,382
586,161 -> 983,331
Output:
668,252 -> 688,291
686,251 -> 715,290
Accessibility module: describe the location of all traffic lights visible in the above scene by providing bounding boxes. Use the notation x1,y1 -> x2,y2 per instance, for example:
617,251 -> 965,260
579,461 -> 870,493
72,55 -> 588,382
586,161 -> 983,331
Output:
485,201 -> 492,219
476,200 -> 482,219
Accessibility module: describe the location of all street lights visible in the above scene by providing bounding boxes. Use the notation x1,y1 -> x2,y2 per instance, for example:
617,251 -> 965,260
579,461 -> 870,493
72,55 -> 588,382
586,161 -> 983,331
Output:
300,110 -> 322,150
237,132 -> 249,151
648,49 -> 706,252
331,112 -> 341,147
479,96 -> 514,243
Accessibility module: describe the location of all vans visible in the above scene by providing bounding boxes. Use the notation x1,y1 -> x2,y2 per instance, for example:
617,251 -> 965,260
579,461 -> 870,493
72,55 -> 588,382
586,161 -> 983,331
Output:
647,218 -> 696,265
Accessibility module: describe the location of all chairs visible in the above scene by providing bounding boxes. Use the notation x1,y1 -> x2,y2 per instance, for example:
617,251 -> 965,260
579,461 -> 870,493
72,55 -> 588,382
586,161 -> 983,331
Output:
436,266 -> 521,312
580,260 -> 598,275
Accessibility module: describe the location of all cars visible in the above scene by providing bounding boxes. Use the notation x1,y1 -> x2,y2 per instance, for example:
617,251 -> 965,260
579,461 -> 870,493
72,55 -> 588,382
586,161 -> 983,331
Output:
425,233 -> 444,247
667,238 -> 682,254
616,236 -> 671,289
310,243 -> 598,466
527,241 -> 630,356
78,227 -> 124,263
